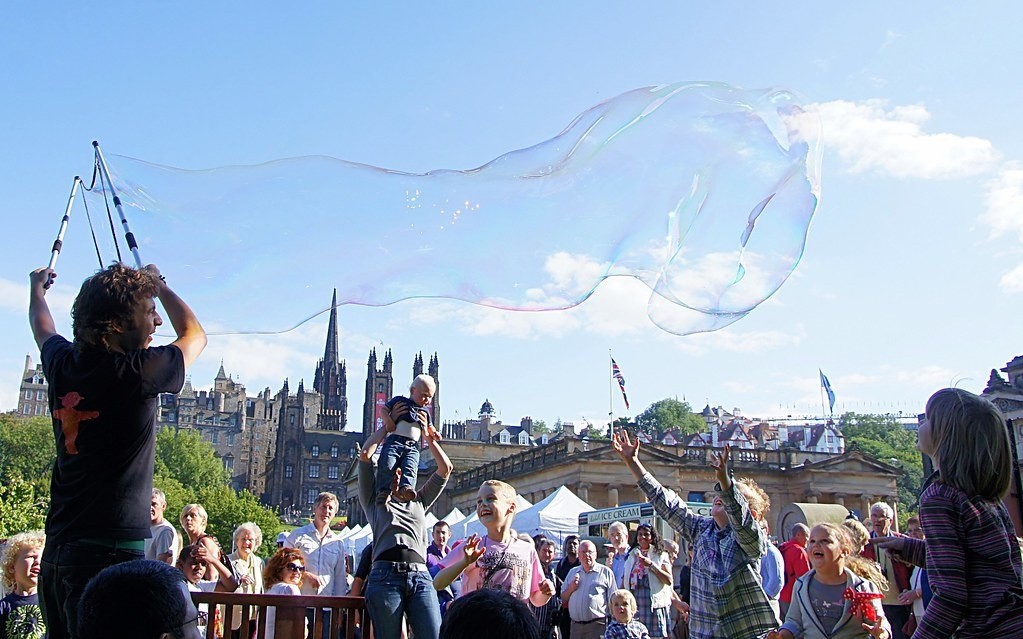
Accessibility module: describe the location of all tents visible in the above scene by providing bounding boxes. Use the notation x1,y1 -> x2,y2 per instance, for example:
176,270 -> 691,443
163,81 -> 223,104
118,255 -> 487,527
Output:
335,485 -> 720,597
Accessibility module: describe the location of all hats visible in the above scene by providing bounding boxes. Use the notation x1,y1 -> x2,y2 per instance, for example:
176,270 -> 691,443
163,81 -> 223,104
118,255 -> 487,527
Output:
276,532 -> 291,542
601,539 -> 614,548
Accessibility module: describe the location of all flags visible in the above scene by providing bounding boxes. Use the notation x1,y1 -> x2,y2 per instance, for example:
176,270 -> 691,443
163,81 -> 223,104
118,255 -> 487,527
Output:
820,370 -> 835,414
612,359 -> 629,410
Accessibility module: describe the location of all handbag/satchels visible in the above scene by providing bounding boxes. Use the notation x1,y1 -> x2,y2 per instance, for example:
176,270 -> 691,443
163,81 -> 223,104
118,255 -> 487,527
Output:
902,610 -> 918,636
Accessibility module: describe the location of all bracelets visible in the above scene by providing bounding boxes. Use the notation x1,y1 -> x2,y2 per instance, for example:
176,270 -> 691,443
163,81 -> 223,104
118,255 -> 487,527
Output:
916,589 -> 921,598
155,274 -> 167,285
910,612 -> 914,615
647,561 -> 653,567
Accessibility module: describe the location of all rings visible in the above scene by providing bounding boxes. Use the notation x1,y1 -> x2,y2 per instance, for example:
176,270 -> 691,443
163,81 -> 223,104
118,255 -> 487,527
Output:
907,600 -> 910,603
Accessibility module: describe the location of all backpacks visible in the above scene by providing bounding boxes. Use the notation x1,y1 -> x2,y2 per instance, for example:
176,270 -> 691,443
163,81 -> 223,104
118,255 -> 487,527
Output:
779,541 -> 800,584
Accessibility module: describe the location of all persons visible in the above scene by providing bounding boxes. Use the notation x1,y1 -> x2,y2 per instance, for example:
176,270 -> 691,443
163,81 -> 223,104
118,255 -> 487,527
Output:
869,387 -> 1023,639
25,265 -> 206,639
433,481 -> 556,607
283,492 -> 347,639
144,487 -> 179,567
0,517 -> 1023,639
858,501 -> 918,639
374,375 -> 444,505
356,402 -> 453,639
611,430 -> 779,639
175,503 -> 223,582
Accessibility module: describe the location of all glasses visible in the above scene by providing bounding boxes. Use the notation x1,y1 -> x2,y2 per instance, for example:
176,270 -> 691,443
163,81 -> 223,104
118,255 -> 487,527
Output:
286,562 -> 306,574
608,533 -> 626,539
714,482 -> 721,491
906,527 -> 922,534
688,546 -> 693,551
181,606 -> 209,627
637,532 -> 651,537
871,513 -> 890,521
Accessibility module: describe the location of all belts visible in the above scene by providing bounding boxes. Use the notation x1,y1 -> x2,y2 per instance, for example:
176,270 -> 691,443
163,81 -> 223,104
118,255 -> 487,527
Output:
573,617 -> 604,624
371,561 -> 428,573
